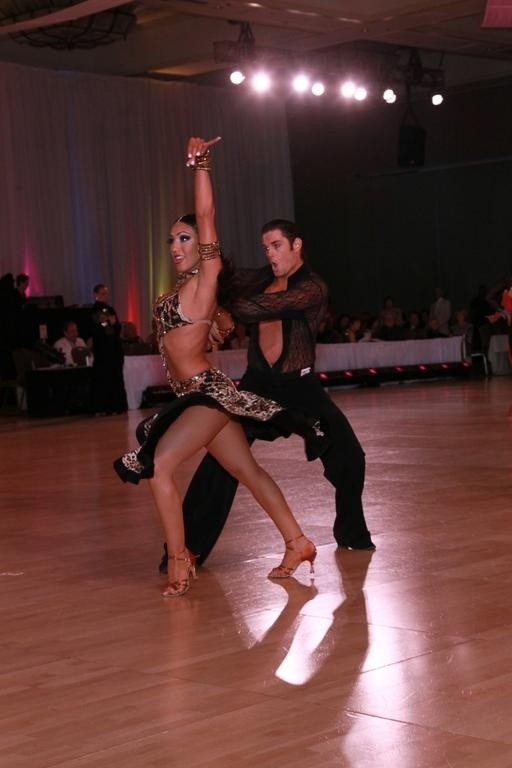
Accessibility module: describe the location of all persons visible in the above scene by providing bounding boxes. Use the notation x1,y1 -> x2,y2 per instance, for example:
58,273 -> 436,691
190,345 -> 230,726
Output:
231,324 -> 249,349
157,219 -> 378,573
113,136 -> 318,596
317,285 -> 512,353
0,267 -> 158,418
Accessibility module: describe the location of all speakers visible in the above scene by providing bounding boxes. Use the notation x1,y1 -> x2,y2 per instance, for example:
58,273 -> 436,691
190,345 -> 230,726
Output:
399,126 -> 425,167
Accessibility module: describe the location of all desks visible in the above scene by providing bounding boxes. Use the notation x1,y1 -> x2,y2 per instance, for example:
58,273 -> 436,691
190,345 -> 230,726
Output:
25,364 -> 93,419
123,335 -> 467,411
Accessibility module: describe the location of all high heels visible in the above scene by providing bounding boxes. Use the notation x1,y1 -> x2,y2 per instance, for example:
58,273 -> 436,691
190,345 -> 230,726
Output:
159,546 -> 200,599
267,533 -> 317,579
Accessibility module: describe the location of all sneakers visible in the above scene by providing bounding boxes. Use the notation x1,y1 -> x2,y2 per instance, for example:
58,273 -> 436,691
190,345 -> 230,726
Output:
346,537 -> 376,551
159,553 -> 169,577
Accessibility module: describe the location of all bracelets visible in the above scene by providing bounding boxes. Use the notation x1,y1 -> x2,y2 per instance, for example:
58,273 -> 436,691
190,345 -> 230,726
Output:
191,155 -> 213,172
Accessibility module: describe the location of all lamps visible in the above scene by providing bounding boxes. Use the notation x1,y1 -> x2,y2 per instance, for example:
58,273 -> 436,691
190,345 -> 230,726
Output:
229,61 -> 446,106
